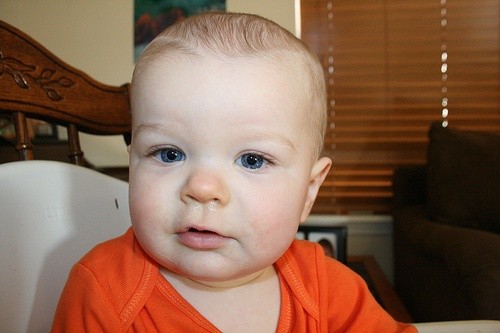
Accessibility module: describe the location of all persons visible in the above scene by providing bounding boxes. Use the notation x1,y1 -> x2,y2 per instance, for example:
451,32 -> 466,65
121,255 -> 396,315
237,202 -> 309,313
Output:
50,10 -> 418,333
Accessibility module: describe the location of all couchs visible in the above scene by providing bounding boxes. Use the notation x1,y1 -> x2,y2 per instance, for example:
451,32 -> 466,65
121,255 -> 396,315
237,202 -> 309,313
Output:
392,163 -> 500,322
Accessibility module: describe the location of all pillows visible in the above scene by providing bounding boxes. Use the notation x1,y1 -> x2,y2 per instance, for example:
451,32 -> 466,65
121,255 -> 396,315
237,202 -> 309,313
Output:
424,120 -> 500,234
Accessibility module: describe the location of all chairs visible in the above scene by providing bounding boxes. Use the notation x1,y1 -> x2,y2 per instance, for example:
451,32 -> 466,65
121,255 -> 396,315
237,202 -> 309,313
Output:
0,18 -> 133,333
